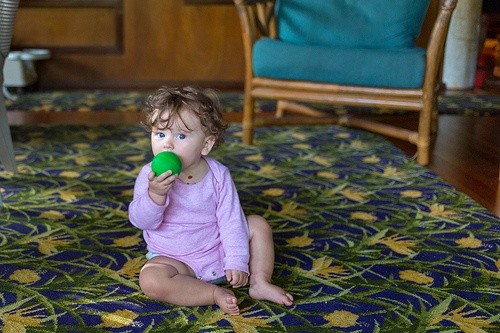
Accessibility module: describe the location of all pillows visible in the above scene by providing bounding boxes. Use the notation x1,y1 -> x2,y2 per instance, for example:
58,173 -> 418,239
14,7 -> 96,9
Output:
272,0 -> 431,48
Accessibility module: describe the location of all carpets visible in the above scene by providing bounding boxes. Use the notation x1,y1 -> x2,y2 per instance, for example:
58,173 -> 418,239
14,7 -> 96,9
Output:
0,123 -> 500,333
4,89 -> 500,116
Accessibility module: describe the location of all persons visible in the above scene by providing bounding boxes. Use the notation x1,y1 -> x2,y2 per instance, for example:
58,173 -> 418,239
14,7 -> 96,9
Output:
129,88 -> 294,315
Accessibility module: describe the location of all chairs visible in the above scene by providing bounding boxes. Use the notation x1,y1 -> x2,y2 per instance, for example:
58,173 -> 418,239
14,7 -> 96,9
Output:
233,0 -> 458,165
